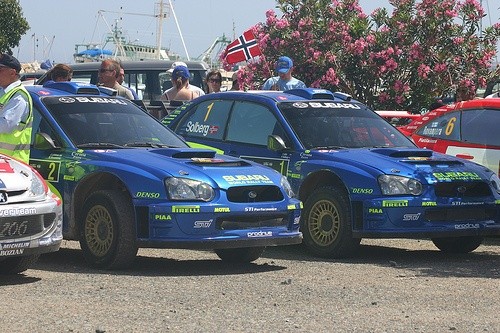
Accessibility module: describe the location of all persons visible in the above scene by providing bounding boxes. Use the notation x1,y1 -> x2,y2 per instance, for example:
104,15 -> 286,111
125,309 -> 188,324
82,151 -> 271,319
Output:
0,54 -> 33,165
90,59 -> 240,101
261,56 -> 307,90
453,84 -> 478,105
48,64 -> 73,81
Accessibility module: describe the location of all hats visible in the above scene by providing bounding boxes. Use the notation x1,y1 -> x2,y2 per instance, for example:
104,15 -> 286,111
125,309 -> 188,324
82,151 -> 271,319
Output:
172,65 -> 190,80
166,61 -> 187,73
208,78 -> 221,83
0,54 -> 21,73
275,57 -> 294,74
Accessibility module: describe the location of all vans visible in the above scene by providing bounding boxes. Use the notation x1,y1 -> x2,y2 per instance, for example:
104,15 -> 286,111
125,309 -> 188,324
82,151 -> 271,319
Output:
30,60 -> 213,115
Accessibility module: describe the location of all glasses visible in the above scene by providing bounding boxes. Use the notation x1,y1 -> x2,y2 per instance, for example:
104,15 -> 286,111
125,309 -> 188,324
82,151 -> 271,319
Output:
98,68 -> 114,73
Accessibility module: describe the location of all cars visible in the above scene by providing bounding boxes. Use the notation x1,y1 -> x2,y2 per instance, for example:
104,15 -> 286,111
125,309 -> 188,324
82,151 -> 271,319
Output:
384,98 -> 500,181
157,88 -> 500,260
0,153 -> 63,275
353,110 -> 422,144
22,81 -> 303,270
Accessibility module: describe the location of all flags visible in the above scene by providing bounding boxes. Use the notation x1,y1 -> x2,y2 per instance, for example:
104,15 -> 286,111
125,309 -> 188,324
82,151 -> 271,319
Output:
225,30 -> 262,64
